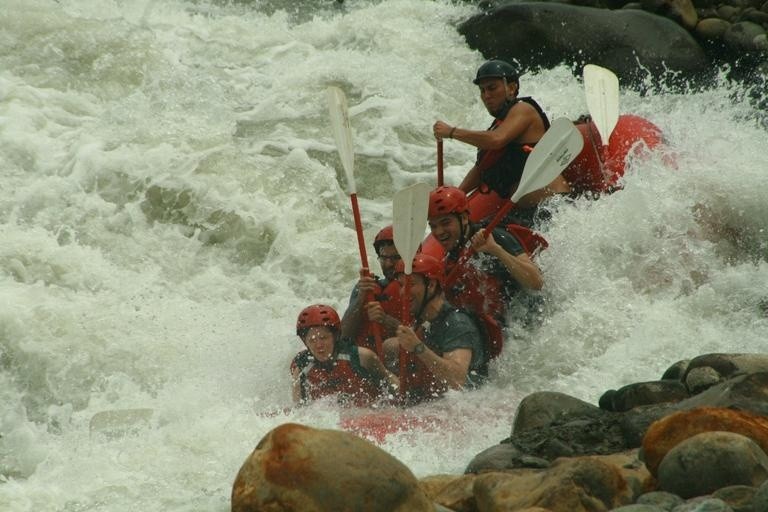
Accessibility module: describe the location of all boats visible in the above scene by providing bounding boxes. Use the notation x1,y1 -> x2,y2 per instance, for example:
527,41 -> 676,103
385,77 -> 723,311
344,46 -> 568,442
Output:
316,113 -> 678,445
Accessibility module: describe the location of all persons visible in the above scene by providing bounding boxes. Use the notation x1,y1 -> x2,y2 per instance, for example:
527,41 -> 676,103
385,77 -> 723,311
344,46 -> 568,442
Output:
380,253 -> 488,412
339,224 -> 422,357
434,61 -> 579,268
291,305 -> 398,418
426,184 -> 547,325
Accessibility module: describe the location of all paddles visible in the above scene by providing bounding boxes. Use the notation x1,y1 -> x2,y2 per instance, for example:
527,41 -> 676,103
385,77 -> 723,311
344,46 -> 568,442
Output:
328,83 -> 385,363
443,117 -> 586,287
392,184 -> 430,406
582,63 -> 620,192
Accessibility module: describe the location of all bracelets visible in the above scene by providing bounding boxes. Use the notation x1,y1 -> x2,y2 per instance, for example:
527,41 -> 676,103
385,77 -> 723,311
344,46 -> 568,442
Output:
449,127 -> 455,138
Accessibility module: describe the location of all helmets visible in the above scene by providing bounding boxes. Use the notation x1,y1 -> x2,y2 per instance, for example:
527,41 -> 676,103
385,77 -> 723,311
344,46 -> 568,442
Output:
472,61 -> 519,84
296,303 -> 341,334
374,226 -> 446,289
428,185 -> 470,218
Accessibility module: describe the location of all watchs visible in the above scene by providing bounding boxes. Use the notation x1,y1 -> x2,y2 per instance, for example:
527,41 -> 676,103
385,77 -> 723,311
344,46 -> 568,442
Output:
415,340 -> 424,354
